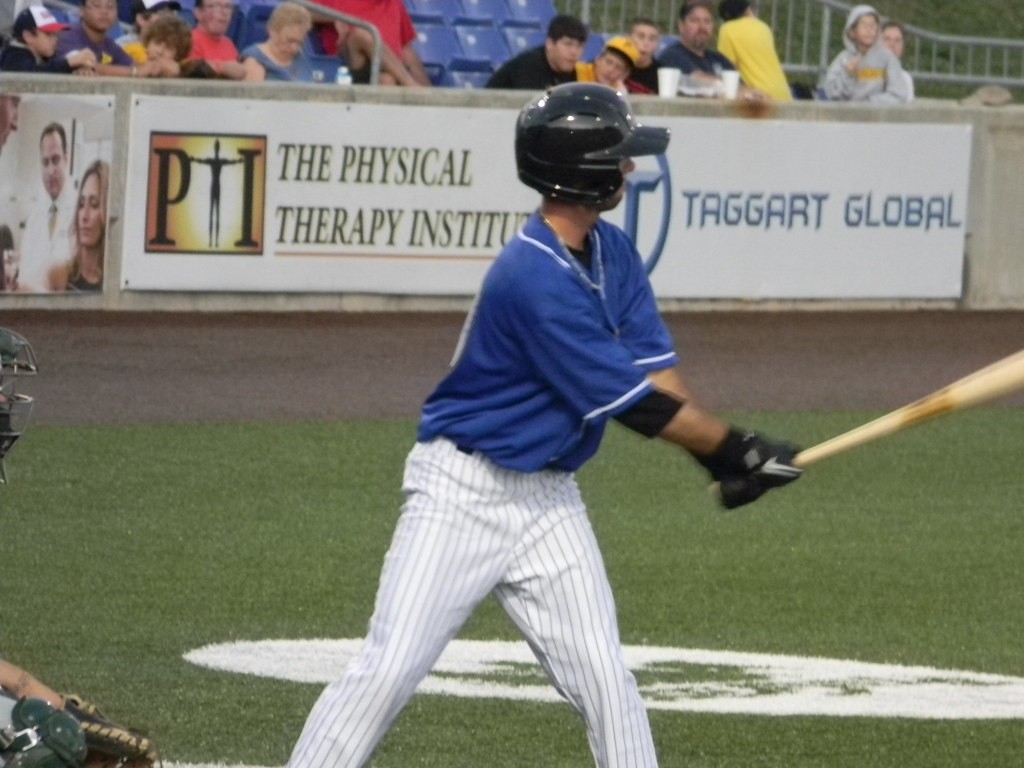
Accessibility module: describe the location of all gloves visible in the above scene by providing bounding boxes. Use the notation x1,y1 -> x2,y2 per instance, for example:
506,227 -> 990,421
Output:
687,423 -> 804,511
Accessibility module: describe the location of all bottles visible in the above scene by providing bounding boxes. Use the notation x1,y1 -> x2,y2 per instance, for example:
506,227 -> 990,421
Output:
335,67 -> 352,85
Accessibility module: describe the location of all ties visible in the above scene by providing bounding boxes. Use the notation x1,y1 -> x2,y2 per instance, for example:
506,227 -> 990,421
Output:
48,203 -> 58,241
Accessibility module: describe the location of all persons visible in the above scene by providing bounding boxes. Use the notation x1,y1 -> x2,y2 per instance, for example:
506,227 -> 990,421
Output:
304,0 -> 431,87
881,22 -> 913,100
38,159 -> 108,290
658,0 -> 770,101
822,5 -> 908,104
576,35 -> 640,94
0,93 -> 77,290
626,18 -> 663,94
0,327 -> 155,768
717,0 -> 794,102
289,82 -> 800,768
0,0 -> 265,80
241,2 -> 314,81
486,15 -> 587,91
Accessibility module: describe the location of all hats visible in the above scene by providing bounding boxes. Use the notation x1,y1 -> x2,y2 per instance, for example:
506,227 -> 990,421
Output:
12,5 -> 73,34
604,35 -> 642,69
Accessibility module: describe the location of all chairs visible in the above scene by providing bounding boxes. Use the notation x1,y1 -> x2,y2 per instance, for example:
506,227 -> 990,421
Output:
44,0 -> 678,88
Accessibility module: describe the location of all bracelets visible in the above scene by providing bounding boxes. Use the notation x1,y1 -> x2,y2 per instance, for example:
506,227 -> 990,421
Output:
132,66 -> 137,77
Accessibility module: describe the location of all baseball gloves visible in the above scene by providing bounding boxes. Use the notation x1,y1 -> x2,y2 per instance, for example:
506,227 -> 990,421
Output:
59,690 -> 166,768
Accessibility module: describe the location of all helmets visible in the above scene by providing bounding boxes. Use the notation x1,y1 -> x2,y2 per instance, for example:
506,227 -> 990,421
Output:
0,325 -> 41,461
514,81 -> 672,206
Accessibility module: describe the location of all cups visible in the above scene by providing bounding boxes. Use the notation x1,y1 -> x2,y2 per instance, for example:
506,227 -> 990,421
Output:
658,67 -> 680,98
721,69 -> 740,98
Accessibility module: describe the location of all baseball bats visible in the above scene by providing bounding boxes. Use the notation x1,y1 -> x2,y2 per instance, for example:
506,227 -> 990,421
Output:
706,352 -> 1024,500
